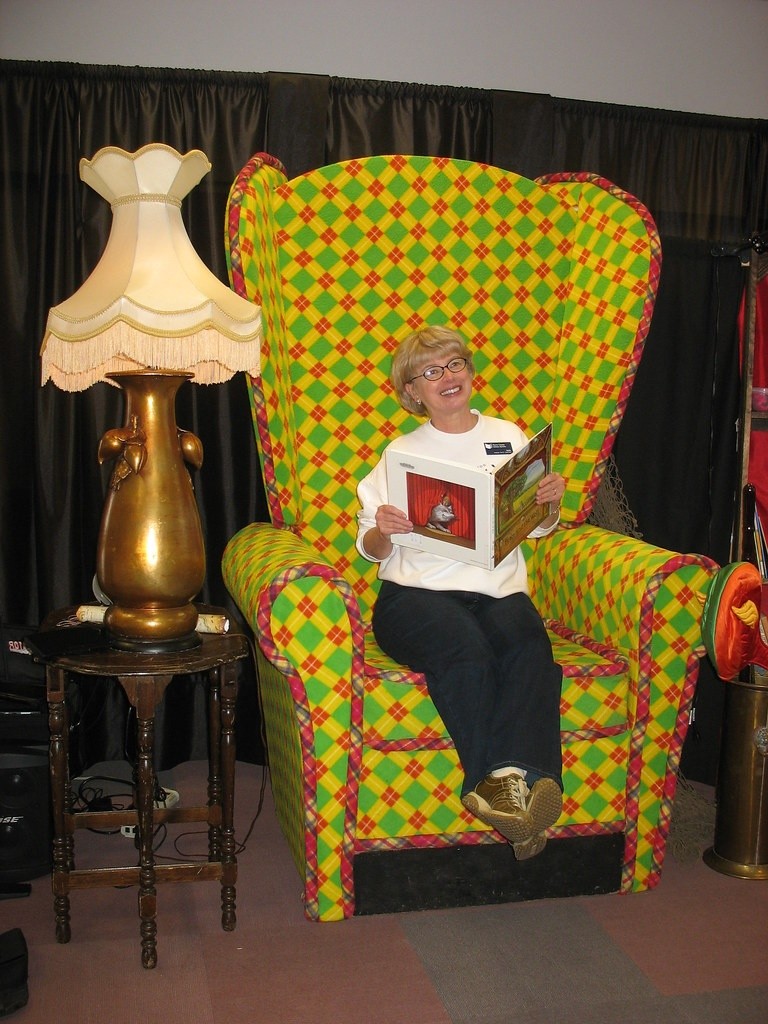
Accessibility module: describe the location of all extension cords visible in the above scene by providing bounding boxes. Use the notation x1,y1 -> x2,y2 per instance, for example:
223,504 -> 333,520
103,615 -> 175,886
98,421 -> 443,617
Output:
120,787 -> 181,839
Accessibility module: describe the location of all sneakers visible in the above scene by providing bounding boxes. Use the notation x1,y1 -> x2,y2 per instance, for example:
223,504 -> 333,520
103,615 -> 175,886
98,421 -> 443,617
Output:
461,774 -> 535,842
508,777 -> 563,861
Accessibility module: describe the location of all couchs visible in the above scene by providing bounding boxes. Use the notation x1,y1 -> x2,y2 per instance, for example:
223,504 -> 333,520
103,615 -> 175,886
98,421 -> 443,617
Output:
221,152 -> 720,922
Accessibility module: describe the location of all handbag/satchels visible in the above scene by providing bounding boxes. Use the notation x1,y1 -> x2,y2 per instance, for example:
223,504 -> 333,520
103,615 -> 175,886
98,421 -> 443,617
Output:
0,623 -> 90,687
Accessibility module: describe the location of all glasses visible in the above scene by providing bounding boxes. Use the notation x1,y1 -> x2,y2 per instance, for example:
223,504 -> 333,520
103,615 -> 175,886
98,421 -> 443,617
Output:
408,357 -> 466,383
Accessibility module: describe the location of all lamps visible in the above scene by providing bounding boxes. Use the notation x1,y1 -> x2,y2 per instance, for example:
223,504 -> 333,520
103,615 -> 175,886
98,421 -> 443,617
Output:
39,141 -> 262,654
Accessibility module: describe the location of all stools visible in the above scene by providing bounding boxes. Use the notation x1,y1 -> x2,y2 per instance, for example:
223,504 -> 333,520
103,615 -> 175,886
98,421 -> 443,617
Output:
39,600 -> 249,969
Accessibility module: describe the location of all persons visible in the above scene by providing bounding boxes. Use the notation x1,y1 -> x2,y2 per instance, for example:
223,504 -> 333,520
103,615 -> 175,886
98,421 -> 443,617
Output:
356,327 -> 565,860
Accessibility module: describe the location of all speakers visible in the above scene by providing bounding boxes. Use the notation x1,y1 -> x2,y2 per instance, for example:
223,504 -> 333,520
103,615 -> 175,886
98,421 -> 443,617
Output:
0,746 -> 55,884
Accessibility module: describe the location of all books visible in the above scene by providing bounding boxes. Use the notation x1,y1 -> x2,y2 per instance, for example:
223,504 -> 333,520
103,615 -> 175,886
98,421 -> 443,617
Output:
384,422 -> 552,572
